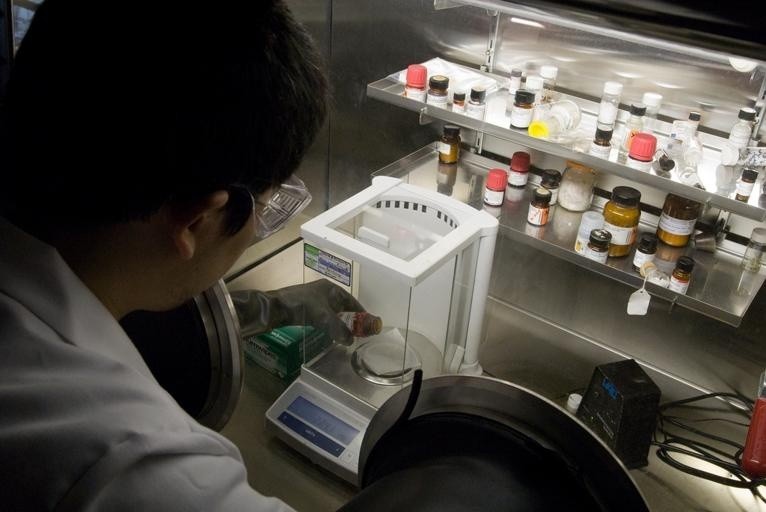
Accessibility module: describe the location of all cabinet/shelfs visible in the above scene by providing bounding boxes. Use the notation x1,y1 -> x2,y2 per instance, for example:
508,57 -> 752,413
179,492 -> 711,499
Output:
366,56 -> 766,435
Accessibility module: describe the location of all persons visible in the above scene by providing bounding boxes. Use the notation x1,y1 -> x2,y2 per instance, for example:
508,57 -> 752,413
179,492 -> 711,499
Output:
0,0 -> 369,512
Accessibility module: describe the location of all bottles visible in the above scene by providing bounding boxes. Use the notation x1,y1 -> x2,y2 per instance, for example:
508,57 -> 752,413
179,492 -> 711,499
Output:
482,169 -> 508,208
508,152 -> 531,189
438,125 -> 463,167
336,310 -> 381,338
742,230 -> 766,269
509,64 -> 761,203
404,63 -> 487,122
527,159 -> 704,295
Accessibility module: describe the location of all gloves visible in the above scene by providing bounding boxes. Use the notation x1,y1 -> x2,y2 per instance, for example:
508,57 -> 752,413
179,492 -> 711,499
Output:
230,278 -> 368,346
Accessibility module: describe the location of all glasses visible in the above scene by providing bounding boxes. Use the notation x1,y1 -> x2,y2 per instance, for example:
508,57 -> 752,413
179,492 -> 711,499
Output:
247,174 -> 312,238
